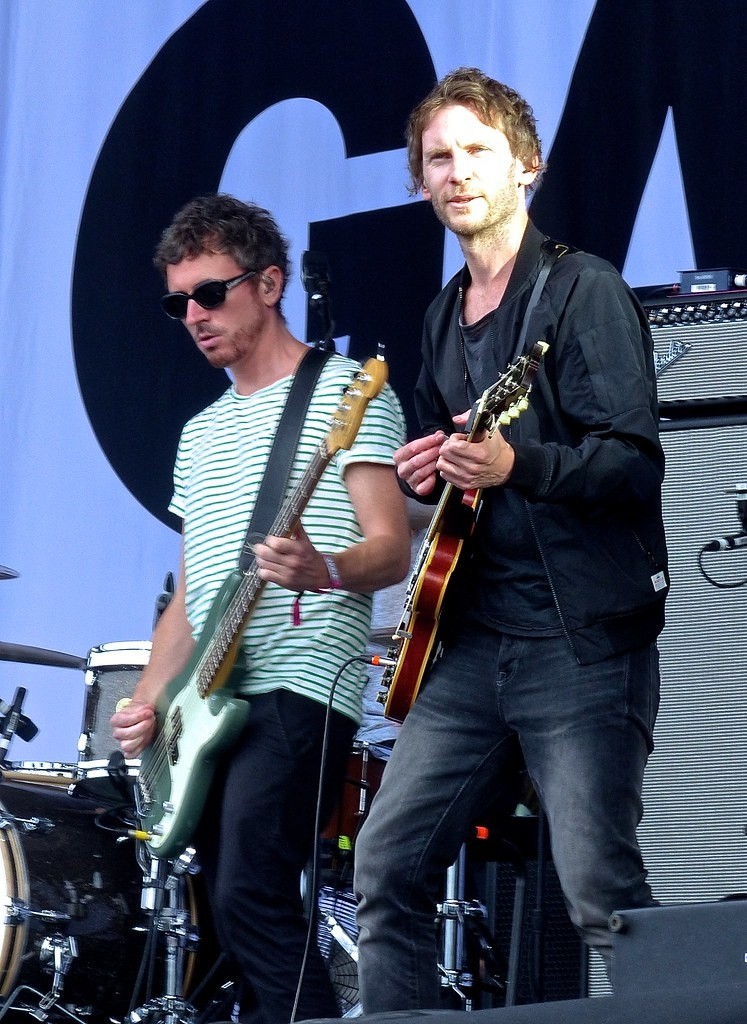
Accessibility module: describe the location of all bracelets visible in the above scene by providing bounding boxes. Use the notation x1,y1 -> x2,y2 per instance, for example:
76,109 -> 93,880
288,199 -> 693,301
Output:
303,555 -> 341,595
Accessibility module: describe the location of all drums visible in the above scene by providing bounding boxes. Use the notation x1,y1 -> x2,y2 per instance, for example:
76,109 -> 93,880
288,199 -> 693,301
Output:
0,786 -> 199,1024
74,638 -> 153,809
1,760 -> 78,783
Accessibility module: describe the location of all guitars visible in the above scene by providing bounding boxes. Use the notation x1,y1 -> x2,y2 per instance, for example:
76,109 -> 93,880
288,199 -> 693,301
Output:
133,349 -> 388,862
376,336 -> 552,725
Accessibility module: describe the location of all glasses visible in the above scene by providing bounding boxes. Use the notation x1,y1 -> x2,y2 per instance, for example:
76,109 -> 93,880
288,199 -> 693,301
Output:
161,269 -> 255,321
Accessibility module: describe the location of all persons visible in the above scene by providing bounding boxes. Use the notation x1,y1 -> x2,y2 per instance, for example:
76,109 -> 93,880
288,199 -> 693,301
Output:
354,73 -> 671,1016
110,198 -> 411,1024
